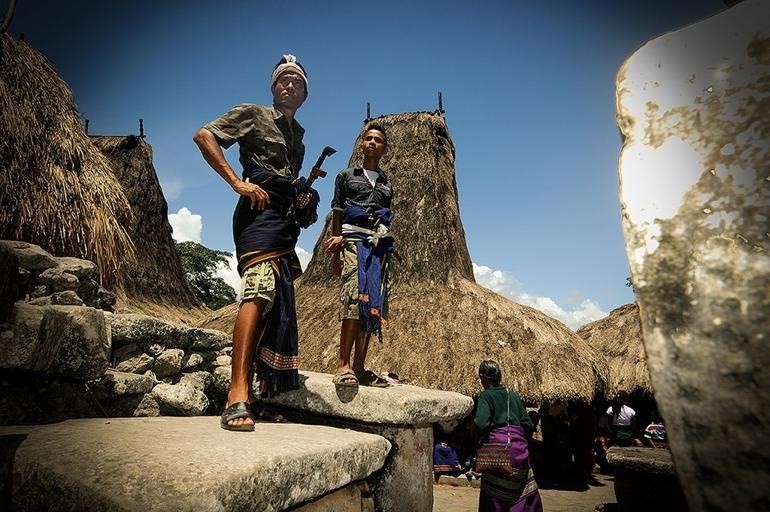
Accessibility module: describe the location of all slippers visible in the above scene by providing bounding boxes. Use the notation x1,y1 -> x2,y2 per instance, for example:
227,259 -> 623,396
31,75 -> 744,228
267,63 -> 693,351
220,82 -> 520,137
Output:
332,370 -> 389,387
220,400 -> 287,431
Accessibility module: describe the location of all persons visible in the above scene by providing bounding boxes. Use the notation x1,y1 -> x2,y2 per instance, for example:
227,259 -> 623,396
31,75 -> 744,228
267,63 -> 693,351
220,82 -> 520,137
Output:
464,361 -> 544,511
434,438 -> 476,475
644,410 -> 669,449
193,55 -> 309,431
604,390 -> 638,446
324,123 -> 394,387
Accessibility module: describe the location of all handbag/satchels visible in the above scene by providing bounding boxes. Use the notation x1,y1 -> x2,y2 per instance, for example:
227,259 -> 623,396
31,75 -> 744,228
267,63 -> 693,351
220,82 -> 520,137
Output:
476,444 -> 512,476
